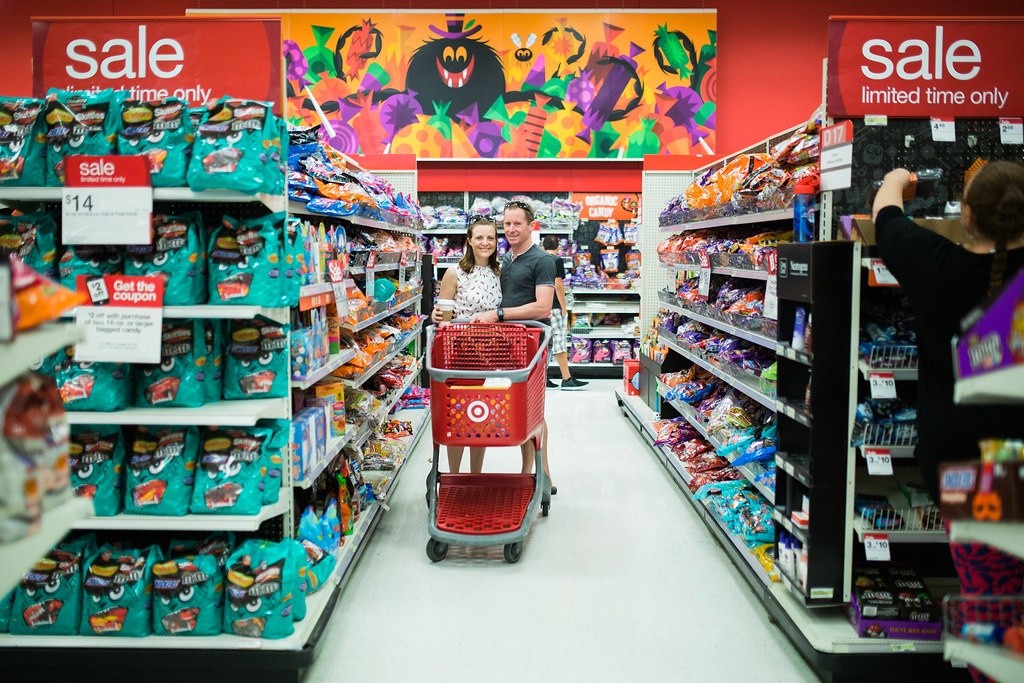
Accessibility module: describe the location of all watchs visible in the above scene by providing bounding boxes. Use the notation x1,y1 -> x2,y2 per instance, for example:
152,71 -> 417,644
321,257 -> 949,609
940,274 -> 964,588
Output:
496,307 -> 505,321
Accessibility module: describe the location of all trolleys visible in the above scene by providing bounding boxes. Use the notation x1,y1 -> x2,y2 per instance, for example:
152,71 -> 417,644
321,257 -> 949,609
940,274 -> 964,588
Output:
423,313 -> 550,560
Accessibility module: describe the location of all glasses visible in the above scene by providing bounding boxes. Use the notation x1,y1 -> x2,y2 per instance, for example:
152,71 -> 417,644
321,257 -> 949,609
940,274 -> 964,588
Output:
469,215 -> 494,224
504,202 -> 534,220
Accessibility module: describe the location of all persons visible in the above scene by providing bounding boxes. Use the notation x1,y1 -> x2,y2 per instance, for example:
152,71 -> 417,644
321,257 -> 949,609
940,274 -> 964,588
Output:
431,216 -> 504,474
542,235 -> 590,390
471,200 -> 557,485
871,160 -> 1022,683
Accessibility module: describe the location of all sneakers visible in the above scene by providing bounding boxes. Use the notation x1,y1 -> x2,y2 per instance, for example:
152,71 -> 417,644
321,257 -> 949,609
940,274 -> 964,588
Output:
545,378 -> 559,389
562,378 -> 590,390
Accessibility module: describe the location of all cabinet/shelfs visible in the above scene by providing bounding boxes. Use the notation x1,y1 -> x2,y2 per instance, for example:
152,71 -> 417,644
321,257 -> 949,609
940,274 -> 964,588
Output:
429,191 -> 642,379
0,85 -> 429,678
628,103 -> 1024,683
